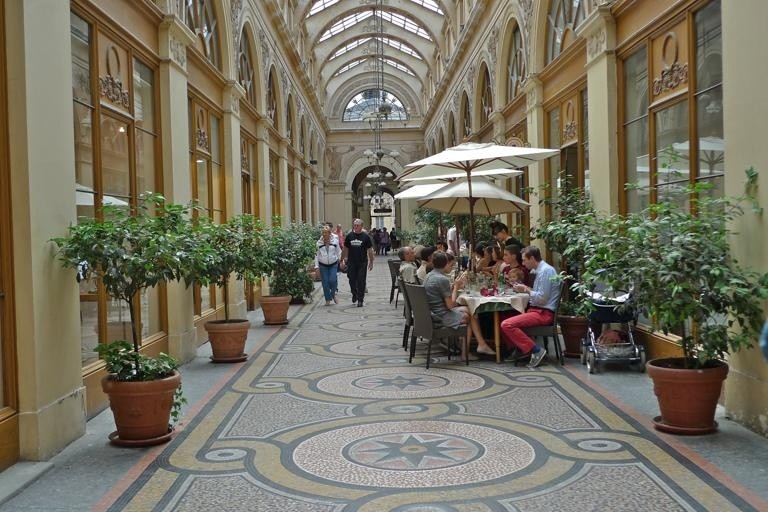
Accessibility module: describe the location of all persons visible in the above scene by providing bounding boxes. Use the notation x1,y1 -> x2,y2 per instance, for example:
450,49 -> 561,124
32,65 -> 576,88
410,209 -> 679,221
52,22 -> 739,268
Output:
314,218 -> 397,307
398,218 -> 561,367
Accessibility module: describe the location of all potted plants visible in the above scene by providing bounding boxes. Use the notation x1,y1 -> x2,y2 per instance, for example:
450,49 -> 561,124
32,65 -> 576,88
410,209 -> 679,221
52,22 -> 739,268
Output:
45,190 -> 232,440
258,215 -> 298,322
583,146 -> 768,427
194,212 -> 272,358
519,170 -> 611,354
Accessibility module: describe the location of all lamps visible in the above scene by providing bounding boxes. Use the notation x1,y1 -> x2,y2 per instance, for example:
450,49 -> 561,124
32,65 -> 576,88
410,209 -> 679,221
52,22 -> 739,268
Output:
362,1 -> 401,208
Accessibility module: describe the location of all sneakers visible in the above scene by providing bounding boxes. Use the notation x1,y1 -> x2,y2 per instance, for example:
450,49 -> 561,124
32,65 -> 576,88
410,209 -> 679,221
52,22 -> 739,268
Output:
351,294 -> 358,303
477,344 -> 497,355
325,299 -> 331,306
357,301 -> 364,307
527,347 -> 547,368
504,349 -> 530,362
462,352 -> 481,361
333,296 -> 339,304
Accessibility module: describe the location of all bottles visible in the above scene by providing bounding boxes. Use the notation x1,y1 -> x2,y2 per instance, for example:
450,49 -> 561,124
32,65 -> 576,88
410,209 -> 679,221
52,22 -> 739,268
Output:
497,269 -> 505,296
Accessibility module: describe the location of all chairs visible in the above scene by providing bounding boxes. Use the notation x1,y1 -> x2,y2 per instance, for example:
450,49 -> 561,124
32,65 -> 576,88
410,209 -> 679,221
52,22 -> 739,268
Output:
387,258 -> 399,304
515,282 -> 564,367
398,275 -> 414,351
412,274 -> 456,357
404,283 -> 468,369
394,262 -> 402,309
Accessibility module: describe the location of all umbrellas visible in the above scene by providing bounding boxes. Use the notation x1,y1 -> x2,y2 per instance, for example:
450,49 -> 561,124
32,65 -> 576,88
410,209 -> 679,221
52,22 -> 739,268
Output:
394,179 -> 459,256
392,141 -> 561,277
416,176 -> 532,273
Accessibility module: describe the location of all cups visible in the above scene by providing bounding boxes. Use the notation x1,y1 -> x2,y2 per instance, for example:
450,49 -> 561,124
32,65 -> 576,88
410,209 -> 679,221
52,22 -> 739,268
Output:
464,277 -> 471,294
